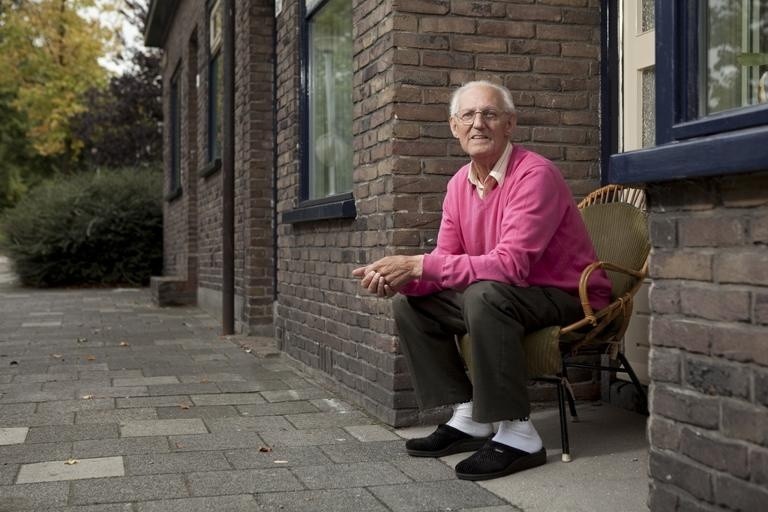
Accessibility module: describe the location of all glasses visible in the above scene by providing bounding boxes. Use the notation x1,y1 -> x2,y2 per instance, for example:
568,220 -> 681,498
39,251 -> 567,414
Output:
454,108 -> 508,125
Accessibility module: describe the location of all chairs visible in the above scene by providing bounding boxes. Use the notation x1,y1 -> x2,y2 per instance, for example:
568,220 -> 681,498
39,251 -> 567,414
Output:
454,184 -> 650,462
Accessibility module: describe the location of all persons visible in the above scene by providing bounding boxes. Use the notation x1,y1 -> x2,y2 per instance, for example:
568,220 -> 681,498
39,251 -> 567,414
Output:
350,80 -> 615,481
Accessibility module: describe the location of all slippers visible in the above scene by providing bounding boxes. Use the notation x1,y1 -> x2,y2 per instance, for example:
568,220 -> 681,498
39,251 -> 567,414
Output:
455,439 -> 547,480
405,423 -> 494,457
